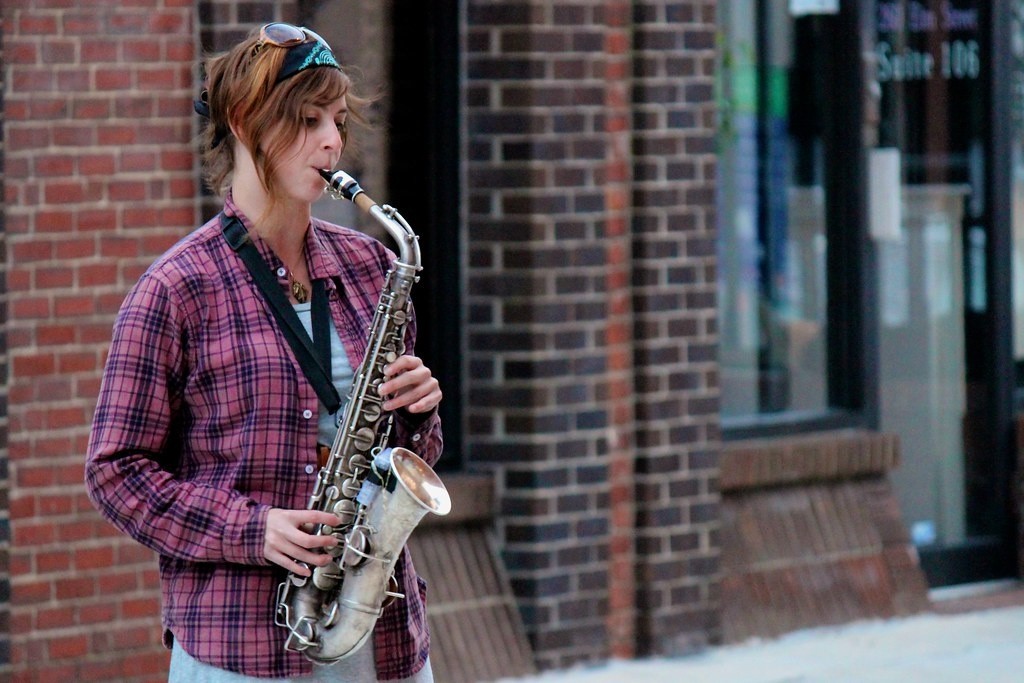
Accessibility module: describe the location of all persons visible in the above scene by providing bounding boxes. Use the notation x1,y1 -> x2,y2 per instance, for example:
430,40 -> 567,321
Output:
82,17 -> 448,681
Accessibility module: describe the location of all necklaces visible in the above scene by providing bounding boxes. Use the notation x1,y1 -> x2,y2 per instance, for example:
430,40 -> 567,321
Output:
262,232 -> 313,304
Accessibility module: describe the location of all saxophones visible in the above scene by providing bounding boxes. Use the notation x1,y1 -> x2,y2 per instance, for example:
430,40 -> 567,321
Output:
272,159 -> 452,668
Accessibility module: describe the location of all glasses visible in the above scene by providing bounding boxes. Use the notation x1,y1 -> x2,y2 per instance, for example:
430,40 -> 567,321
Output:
251,21 -> 335,60
